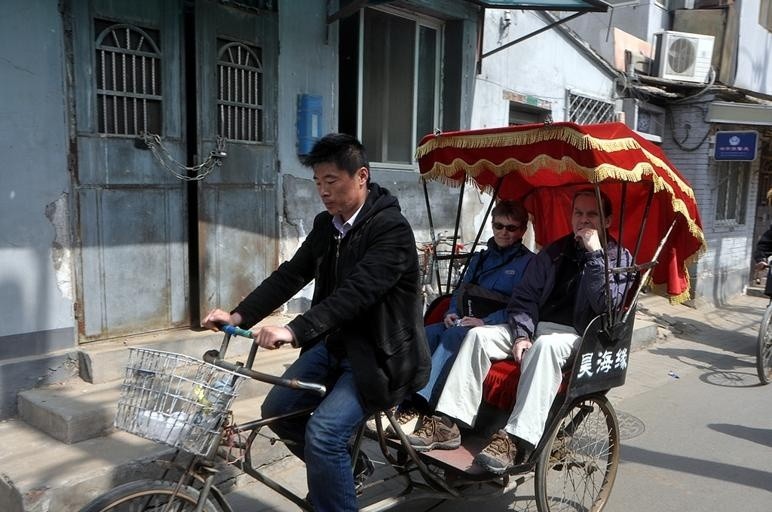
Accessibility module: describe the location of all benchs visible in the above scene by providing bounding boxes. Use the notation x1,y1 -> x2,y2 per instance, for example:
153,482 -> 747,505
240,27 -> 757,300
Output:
420,272 -> 638,414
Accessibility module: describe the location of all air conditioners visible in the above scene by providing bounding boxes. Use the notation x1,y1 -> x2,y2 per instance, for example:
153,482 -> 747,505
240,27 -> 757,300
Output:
648,31 -> 716,84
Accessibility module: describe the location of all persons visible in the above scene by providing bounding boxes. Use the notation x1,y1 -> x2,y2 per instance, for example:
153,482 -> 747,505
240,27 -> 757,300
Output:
364,198 -> 537,439
201,132 -> 434,512
405,188 -> 634,474
753,224 -> 771,308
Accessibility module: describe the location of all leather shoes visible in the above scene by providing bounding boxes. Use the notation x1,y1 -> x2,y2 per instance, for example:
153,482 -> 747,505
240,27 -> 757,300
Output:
474,428 -> 535,474
305,450 -> 374,504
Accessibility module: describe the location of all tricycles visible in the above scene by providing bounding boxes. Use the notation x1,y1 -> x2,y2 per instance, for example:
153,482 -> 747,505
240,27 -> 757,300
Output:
75,122 -> 706,512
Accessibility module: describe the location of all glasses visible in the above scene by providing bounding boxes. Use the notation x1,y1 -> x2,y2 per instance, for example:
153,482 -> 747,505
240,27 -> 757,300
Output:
492,222 -> 521,232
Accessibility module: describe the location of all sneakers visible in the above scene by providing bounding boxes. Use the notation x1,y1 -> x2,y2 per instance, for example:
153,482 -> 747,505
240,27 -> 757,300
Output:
365,406 -> 462,451
